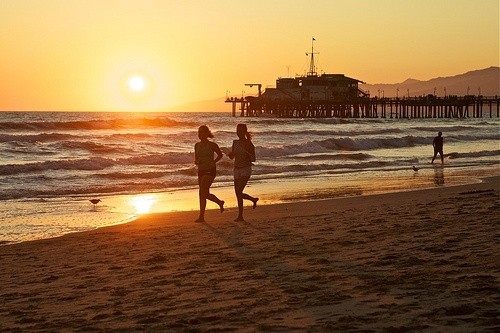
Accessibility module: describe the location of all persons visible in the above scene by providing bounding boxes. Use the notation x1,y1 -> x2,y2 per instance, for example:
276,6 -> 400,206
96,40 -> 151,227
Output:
431,131 -> 445,164
194,124 -> 225,222
219,122 -> 259,222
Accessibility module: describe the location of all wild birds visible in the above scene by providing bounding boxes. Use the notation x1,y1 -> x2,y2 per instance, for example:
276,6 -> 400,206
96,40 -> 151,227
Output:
89,198 -> 102,206
412,166 -> 419,173
